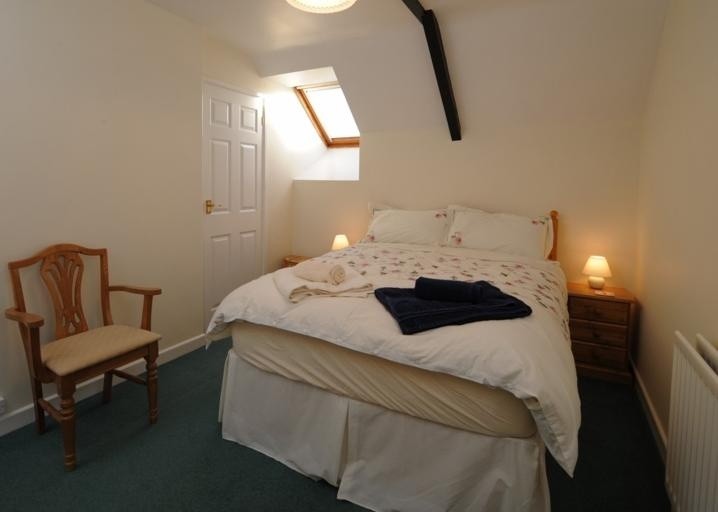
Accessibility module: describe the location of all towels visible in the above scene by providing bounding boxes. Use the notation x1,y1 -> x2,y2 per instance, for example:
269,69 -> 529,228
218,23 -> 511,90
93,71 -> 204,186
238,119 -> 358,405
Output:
271,260 -> 375,304
374,276 -> 533,335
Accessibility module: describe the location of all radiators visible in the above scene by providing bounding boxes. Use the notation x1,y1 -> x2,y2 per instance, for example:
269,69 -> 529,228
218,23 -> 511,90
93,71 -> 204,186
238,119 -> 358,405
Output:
663,329 -> 718,511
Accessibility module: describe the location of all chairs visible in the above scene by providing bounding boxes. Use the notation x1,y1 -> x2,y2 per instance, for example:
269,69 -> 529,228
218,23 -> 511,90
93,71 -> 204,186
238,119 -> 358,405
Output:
4,243 -> 163,473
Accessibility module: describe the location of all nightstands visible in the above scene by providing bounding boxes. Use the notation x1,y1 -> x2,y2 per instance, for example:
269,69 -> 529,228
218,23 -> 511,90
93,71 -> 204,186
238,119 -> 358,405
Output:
566,281 -> 638,387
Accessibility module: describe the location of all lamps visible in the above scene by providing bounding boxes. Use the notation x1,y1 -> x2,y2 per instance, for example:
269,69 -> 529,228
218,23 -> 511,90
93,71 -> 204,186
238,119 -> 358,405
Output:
332,235 -> 350,251
286,1 -> 462,142
581,254 -> 613,290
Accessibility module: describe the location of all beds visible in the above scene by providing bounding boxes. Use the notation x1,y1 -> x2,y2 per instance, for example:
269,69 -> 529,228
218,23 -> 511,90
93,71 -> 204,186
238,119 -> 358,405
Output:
223,209 -> 572,511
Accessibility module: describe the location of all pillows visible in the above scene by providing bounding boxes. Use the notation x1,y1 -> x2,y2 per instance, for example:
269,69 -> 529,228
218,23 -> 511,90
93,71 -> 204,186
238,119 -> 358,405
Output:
365,205 -> 553,260
